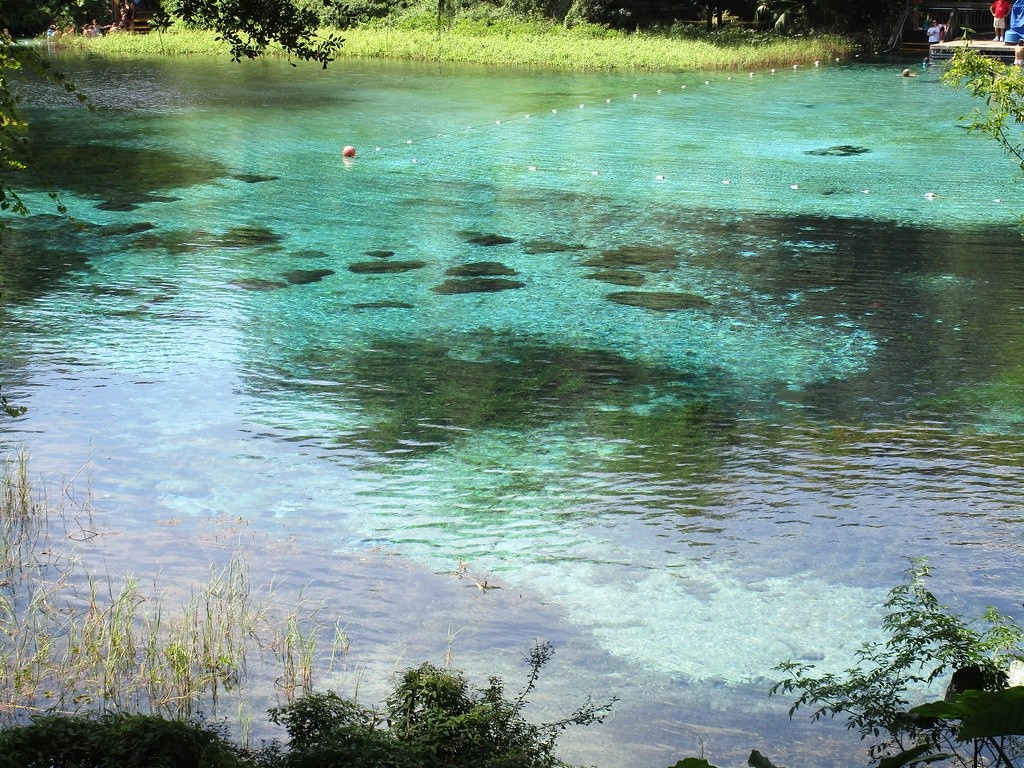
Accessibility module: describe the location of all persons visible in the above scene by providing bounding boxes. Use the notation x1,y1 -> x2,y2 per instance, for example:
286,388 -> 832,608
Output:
902,67 -> 917,77
989,0 -> 1010,42
927,20 -> 945,50
1013,39 -> 1024,68
921,58 -> 937,67
44,4 -> 127,39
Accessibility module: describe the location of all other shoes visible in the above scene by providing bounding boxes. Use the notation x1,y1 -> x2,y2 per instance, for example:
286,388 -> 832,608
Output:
994,37 -> 999,42
1000,37 -> 1004,42
